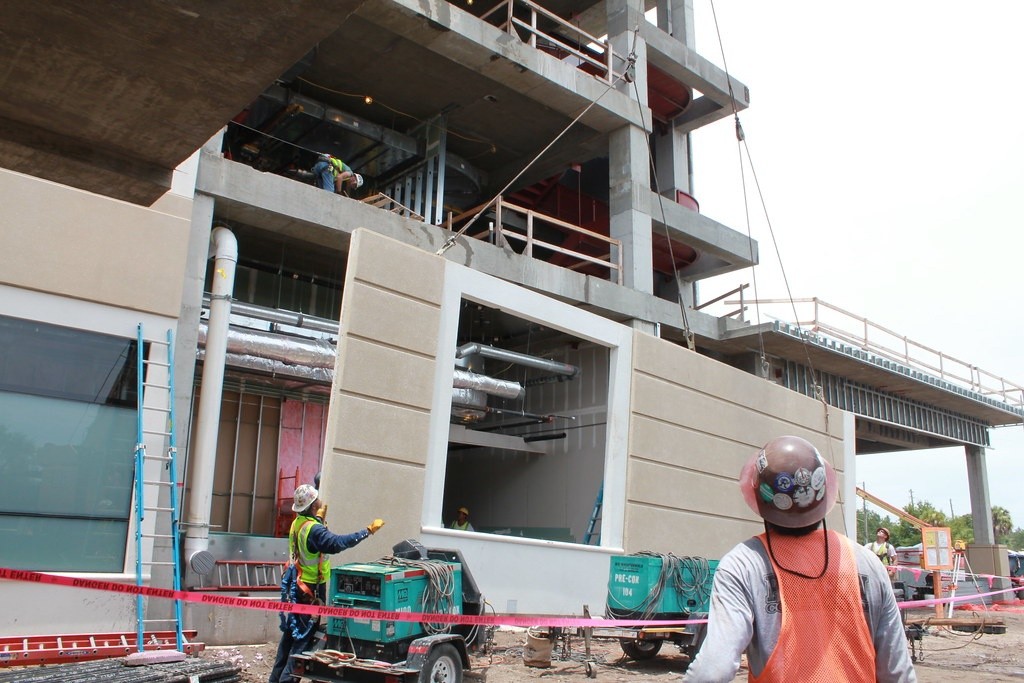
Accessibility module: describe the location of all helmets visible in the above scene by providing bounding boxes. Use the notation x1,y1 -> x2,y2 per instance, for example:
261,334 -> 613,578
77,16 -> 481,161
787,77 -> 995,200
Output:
739,437 -> 837,528
457,507 -> 469,517
291,484 -> 318,512
352,172 -> 363,191
877,527 -> 890,540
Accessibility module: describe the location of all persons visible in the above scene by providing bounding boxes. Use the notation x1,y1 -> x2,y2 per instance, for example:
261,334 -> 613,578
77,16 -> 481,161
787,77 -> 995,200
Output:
268,484 -> 385,683
449,507 -> 475,532
682,436 -> 918,683
862,527 -> 899,581
312,152 -> 363,196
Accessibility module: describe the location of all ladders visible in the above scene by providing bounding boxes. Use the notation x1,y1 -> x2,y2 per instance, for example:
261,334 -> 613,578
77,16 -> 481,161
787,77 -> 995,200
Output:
135,320 -> 184,656
0,628 -> 206,668
185,549 -> 291,592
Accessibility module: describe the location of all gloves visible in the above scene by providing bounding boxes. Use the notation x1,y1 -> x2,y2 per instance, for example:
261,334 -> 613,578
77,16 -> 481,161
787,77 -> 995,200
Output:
317,504 -> 327,517
367,518 -> 385,533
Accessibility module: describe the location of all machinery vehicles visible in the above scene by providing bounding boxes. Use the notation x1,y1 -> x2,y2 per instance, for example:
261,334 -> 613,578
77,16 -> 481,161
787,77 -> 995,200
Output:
604,550 -> 730,669
289,537 -> 506,682
